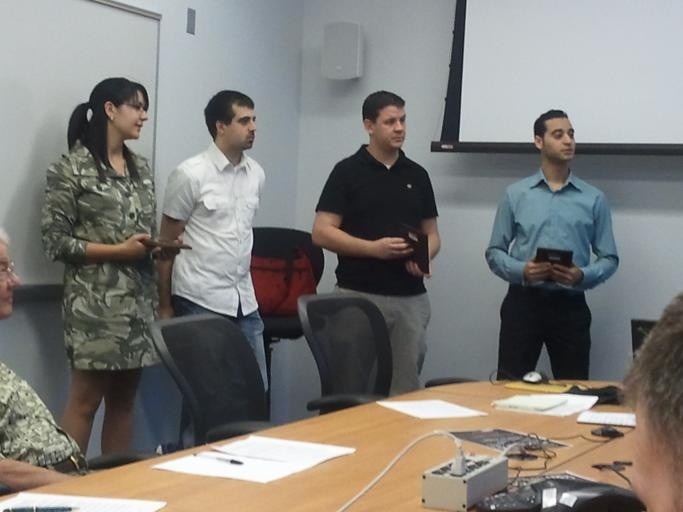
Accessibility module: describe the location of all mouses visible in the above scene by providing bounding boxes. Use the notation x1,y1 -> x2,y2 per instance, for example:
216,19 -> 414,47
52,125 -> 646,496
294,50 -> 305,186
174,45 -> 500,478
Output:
523,370 -> 543,384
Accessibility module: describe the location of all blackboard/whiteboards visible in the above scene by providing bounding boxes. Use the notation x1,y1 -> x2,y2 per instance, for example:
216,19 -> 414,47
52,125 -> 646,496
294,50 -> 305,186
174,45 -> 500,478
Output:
0,0 -> 162,301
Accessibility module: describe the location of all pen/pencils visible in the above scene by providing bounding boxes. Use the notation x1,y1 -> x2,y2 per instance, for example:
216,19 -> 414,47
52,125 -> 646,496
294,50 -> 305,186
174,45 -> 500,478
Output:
2,506 -> 72,512
194,453 -> 244,465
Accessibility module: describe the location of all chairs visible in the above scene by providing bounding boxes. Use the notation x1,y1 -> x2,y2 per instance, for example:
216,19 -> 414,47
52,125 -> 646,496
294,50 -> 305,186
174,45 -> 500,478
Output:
297,292 -> 480,416
0,452 -> 154,494
147,313 -> 277,451
631,319 -> 658,362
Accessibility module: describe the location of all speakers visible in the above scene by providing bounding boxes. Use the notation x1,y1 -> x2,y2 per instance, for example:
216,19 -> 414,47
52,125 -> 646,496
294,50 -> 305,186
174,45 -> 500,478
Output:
321,20 -> 361,82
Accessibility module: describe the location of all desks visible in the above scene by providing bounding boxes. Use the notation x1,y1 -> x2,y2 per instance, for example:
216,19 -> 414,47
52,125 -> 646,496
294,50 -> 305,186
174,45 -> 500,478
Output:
0,381 -> 638,512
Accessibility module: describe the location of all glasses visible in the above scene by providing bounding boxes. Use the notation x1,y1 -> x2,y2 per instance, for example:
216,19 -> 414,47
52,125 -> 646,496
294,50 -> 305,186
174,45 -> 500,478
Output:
121,102 -> 148,111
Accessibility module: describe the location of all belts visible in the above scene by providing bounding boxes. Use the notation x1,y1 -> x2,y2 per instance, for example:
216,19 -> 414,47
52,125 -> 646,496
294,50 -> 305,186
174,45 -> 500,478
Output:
42,452 -> 87,473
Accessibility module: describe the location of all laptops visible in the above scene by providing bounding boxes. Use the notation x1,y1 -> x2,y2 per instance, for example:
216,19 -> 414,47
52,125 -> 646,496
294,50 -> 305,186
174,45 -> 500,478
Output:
629,318 -> 659,362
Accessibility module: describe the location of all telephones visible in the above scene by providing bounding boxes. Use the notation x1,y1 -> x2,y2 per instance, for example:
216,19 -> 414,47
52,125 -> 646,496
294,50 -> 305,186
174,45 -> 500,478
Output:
474,473 -> 648,512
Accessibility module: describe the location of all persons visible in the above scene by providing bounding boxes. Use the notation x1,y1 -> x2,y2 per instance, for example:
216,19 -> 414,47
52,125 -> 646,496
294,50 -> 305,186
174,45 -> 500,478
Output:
156,90 -> 270,454
0,224 -> 90,497
484,108 -> 620,380
614,290 -> 683,512
43,75 -> 185,463
309,89 -> 443,399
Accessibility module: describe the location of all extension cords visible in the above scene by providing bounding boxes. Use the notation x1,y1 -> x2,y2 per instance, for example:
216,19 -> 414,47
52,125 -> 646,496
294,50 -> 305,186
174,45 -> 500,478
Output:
422,451 -> 510,511
